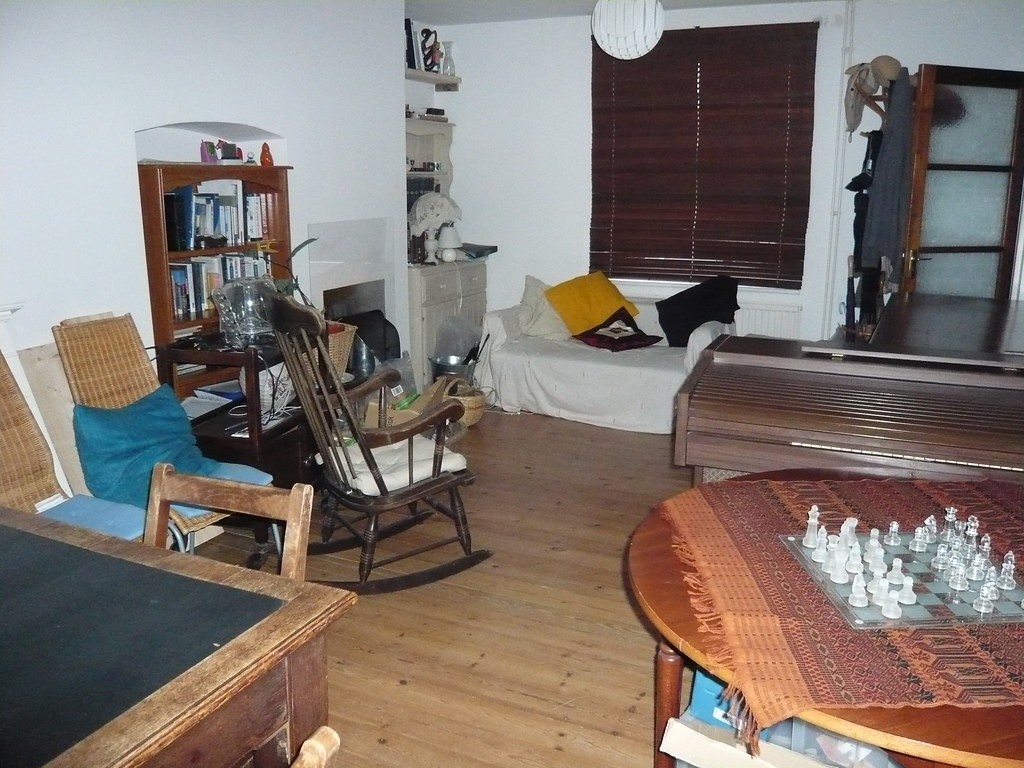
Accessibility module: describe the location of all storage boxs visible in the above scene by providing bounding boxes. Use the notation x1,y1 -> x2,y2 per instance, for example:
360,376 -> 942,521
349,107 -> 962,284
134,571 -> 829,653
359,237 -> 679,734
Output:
690,668 -> 781,743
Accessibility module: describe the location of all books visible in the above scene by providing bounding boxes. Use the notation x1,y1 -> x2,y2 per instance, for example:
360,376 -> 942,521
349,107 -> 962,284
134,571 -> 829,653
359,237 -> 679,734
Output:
405,17 -> 425,70
169,251 -> 272,320
176,364 -> 206,375
164,179 -> 270,250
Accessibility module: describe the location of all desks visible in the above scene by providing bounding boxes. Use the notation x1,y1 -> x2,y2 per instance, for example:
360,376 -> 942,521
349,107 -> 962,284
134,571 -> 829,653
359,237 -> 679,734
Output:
0,506 -> 358,768
628,466 -> 1024,768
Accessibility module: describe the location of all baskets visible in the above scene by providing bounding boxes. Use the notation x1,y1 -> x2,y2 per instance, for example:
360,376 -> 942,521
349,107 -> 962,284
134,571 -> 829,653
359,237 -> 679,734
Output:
302,320 -> 358,388
442,378 -> 486,427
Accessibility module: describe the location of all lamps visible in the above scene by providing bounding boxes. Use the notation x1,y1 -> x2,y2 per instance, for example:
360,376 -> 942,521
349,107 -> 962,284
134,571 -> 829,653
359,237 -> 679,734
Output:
592,0 -> 665,61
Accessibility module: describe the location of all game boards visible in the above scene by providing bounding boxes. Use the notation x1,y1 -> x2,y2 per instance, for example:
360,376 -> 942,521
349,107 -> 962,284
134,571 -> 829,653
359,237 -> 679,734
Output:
775,528 -> 1024,632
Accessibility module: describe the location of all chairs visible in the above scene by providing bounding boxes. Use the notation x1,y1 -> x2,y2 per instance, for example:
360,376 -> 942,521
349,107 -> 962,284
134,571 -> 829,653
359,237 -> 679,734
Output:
256,283 -> 495,597
0,312 -> 313,581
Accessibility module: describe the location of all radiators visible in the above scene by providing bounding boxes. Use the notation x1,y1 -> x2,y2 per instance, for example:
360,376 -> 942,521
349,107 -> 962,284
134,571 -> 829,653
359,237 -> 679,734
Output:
732,304 -> 803,336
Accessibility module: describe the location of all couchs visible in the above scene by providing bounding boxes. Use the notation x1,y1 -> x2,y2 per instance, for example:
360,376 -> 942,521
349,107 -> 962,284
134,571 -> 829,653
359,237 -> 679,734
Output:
470,296 -> 737,435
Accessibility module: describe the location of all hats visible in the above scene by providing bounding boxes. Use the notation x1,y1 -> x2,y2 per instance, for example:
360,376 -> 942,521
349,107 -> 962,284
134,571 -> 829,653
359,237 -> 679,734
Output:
844,55 -> 919,88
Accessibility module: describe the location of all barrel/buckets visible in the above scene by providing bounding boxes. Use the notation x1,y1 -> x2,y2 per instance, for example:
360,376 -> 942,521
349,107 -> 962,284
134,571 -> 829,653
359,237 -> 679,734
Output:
429,355 -> 477,392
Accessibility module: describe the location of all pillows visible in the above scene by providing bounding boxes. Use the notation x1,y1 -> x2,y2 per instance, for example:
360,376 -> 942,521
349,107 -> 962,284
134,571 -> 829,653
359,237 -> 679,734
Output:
72,384 -> 221,512
517,270 -> 664,353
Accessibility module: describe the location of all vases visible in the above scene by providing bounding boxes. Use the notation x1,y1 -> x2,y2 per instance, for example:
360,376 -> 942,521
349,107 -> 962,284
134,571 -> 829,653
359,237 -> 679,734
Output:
442,41 -> 455,77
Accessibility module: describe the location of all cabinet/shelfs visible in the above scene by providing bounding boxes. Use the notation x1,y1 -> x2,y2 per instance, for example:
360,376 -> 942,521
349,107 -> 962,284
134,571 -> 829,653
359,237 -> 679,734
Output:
137,161 -> 345,546
406,117 -> 488,393
